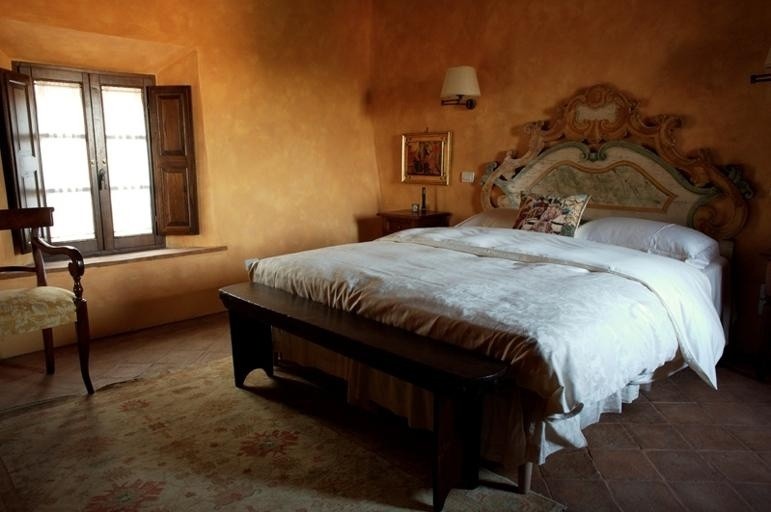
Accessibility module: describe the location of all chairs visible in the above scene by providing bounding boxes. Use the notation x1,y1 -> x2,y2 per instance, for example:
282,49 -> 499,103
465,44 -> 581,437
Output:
0,208 -> 95,396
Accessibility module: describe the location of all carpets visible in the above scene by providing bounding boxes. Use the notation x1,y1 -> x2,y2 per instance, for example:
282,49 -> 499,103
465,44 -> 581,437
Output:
0,348 -> 566,511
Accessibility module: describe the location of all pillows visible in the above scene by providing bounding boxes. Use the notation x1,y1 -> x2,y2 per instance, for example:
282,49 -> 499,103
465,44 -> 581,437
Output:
512,190 -> 591,236
572,213 -> 721,264
454,207 -> 519,228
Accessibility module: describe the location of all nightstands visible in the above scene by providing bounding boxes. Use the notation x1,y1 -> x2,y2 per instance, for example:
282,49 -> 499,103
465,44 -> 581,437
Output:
376,209 -> 451,235
756,248 -> 771,383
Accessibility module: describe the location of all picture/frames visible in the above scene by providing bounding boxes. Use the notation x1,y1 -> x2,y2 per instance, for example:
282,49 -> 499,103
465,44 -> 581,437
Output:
400,130 -> 453,186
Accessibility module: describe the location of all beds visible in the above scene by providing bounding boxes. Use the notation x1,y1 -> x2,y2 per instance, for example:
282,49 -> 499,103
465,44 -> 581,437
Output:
243,80 -> 753,496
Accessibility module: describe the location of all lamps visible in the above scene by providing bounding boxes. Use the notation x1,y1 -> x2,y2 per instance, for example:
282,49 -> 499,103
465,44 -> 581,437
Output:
440,66 -> 481,109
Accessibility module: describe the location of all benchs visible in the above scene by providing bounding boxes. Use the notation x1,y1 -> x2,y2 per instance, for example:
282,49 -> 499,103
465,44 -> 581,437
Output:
217,283 -> 509,512
